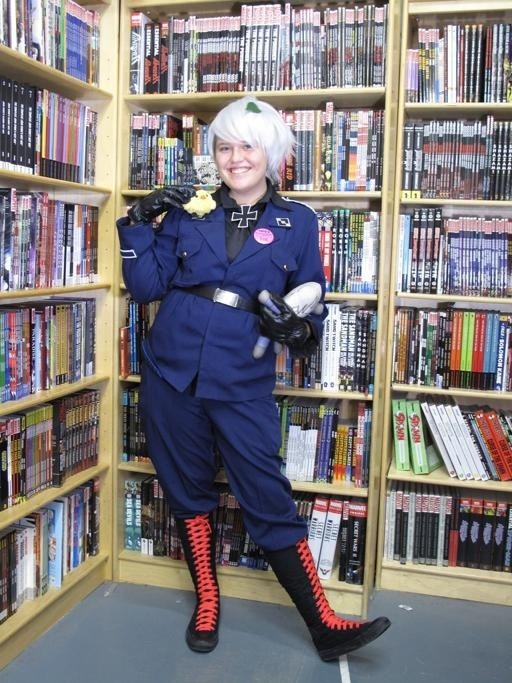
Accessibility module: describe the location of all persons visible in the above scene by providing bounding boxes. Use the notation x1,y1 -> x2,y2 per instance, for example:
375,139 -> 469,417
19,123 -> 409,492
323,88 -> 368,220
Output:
116,95 -> 392,663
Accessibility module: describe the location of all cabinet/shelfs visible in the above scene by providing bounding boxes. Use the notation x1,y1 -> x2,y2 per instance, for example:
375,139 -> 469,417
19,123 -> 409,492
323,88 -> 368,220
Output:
0,0 -> 121,675
374,0 -> 512,609
112,0 -> 403,622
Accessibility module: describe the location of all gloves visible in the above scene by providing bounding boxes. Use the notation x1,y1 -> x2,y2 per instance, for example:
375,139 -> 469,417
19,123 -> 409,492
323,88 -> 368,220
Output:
260,296 -> 308,348
127,188 -> 195,225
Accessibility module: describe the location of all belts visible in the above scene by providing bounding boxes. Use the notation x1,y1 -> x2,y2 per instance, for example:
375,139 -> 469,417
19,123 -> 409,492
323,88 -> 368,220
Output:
172,283 -> 262,315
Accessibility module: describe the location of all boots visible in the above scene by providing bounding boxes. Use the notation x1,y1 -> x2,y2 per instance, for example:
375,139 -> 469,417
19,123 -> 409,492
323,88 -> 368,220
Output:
265,537 -> 392,662
174,512 -> 223,651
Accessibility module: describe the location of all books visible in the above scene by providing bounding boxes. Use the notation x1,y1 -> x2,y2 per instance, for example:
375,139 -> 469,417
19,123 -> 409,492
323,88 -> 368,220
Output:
120,384 -> 150,468
385,486 -> 511,573
125,107 -> 209,187
317,205 -> 383,293
276,100 -> 385,193
2,0 -> 104,89
0,475 -> 101,627
0,74 -> 98,186
276,396 -> 372,490
0,186 -> 98,295
403,111 -> 510,203
0,389 -> 101,515
128,2 -> 387,94
403,16 -> 512,105
119,300 -> 160,373
391,393 -> 512,484
275,302 -> 376,395
209,485 -> 269,572
0,298 -> 96,404
120,474 -> 184,564
293,494 -> 362,587
399,206 -> 512,301
394,308 -> 512,397
